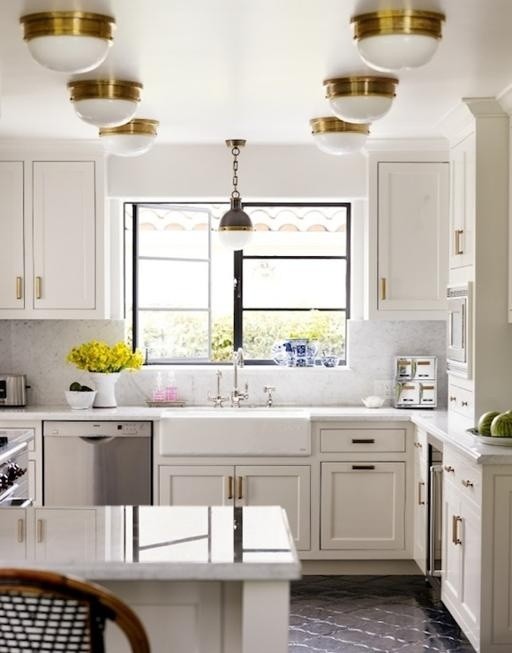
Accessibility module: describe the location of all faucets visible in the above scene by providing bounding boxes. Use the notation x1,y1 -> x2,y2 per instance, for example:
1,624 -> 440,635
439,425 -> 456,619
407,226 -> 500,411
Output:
206,368 -> 230,408
229,347 -> 249,410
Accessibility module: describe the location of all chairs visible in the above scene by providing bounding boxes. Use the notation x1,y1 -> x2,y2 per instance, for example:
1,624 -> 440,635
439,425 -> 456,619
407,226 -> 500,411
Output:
1,569 -> 151,653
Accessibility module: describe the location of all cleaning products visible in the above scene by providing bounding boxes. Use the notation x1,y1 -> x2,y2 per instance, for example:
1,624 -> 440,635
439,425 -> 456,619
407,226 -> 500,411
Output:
152,373 -> 164,401
165,372 -> 178,402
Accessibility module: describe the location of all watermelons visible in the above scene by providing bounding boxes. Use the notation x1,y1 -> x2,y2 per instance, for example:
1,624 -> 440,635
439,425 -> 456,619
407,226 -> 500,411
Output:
489,409 -> 512,440
477,410 -> 500,437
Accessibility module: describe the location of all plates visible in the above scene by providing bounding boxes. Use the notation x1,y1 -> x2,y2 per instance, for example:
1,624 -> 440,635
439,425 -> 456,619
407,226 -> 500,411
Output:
144,400 -> 186,407
467,427 -> 512,446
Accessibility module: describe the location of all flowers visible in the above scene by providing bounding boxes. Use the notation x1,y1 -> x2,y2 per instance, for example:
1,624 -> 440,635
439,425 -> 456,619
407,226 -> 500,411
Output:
66,341 -> 143,374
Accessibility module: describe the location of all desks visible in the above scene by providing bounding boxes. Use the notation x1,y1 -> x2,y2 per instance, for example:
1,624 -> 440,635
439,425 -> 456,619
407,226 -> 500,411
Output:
1,505 -> 303,653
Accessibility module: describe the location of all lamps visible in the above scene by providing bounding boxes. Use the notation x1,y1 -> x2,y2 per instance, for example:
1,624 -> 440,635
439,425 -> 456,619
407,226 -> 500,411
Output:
217,140 -> 253,251
18,9 -> 117,73
98,117 -> 160,158
310,115 -> 371,156
351,9 -> 446,72
324,75 -> 400,125
67,79 -> 144,129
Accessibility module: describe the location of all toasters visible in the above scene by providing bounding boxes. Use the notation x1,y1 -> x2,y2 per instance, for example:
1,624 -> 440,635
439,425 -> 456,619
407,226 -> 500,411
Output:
0,373 -> 31,409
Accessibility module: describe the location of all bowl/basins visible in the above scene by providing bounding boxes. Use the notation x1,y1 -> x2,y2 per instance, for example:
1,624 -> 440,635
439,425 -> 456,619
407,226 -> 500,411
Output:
64,389 -> 98,410
360,396 -> 385,408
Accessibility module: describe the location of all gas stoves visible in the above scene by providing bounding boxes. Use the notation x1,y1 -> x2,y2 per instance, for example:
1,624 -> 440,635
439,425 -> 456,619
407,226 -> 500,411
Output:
0,426 -> 34,507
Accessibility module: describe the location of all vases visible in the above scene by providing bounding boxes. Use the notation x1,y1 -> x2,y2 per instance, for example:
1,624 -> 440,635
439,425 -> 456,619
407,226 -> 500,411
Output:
88,371 -> 122,409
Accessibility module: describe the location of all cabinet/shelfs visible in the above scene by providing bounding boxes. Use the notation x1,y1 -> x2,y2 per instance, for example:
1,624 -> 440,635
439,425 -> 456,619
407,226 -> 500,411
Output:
364,139 -> 451,322
447,383 -> 476,422
446,131 -> 477,290
441,454 -> 479,631
413,425 -> 430,578
151,458 -> 315,576
1,504 -> 112,563
1,135 -> 110,322
316,417 -> 413,575
